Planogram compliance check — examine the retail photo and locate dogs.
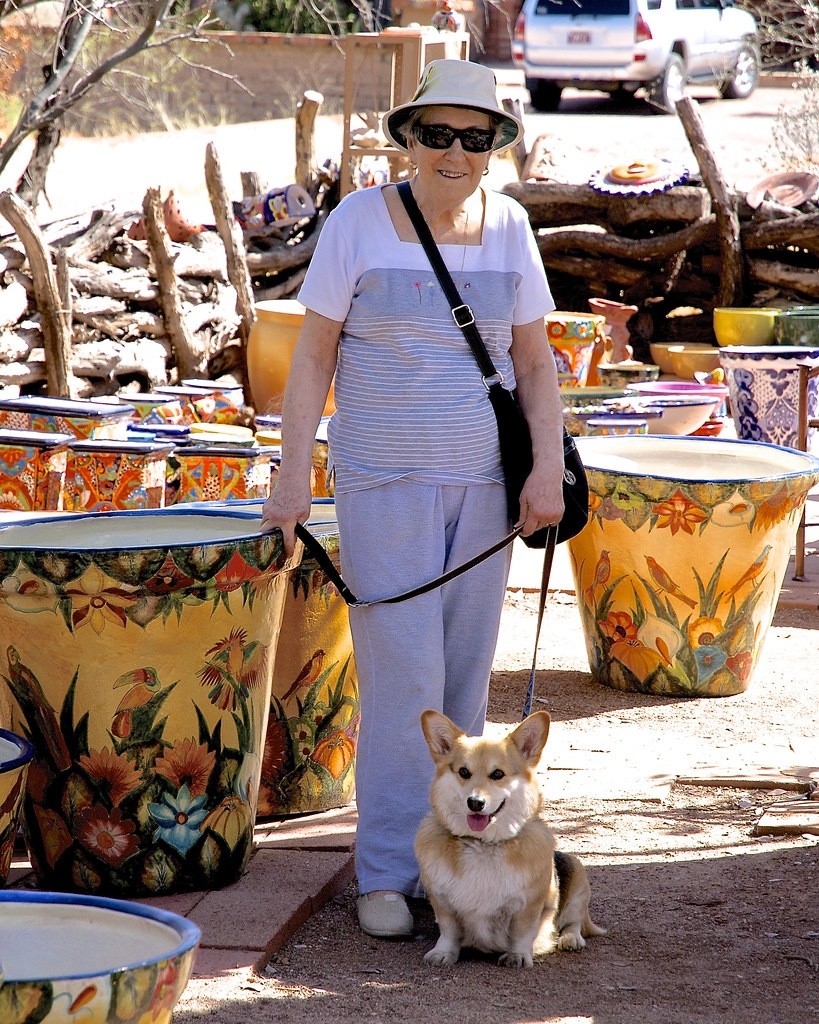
[414,710,607,970]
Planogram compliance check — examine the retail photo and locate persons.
[259,59,564,935]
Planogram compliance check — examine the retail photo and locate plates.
[746,170,819,210]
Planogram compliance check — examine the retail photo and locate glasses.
[412,123,497,153]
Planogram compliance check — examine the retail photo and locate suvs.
[510,0,761,113]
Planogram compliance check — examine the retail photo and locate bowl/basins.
[561,342,729,437]
[774,311,819,347]
[713,308,782,346]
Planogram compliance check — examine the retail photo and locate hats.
[381,58,526,157]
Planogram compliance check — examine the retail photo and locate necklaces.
[409,175,470,297]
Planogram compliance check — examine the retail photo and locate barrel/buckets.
[163,499,361,816]
[566,434,819,698]
[0,890,202,1024]
[0,728,33,890]
[719,346,819,453]
[0,509,308,899]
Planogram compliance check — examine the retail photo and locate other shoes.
[356,893,414,938]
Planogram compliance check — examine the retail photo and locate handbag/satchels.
[486,384,590,549]
